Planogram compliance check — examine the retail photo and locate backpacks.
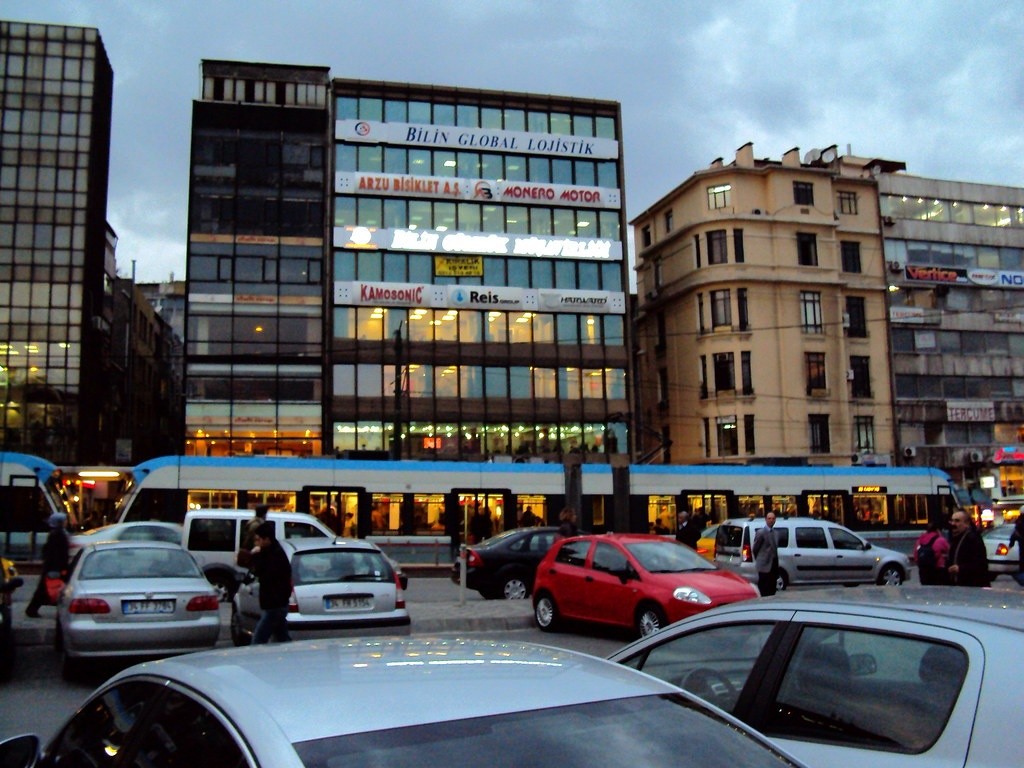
[916,535,939,571]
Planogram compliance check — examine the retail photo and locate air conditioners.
[852,453,862,465]
[904,446,916,457]
[752,208,765,215]
[970,451,983,462]
[842,314,850,327]
[886,214,896,225]
[846,369,854,380]
[891,261,904,270]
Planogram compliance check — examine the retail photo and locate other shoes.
[25,609,41,618]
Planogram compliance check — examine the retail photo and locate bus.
[0,447,975,578]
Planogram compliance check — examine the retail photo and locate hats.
[48,511,68,527]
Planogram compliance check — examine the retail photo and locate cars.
[66,520,188,566]
[532,534,764,641]
[452,525,585,603]
[0,632,814,768]
[56,538,221,667]
[983,523,1024,590]
[229,540,413,647]
[606,585,1022,768]
[696,514,917,594]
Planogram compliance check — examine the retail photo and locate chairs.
[875,644,967,746]
[786,645,859,741]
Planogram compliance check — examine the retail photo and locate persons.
[25,512,72,618]
[914,518,951,585]
[468,507,494,545]
[693,500,883,529]
[516,506,545,527]
[650,518,677,535]
[675,511,701,552]
[244,504,272,586]
[752,511,779,597]
[248,520,293,645]
[1006,505,1024,585]
[446,521,461,564]
[60,470,133,530]
[945,508,991,587]
[554,506,579,544]
